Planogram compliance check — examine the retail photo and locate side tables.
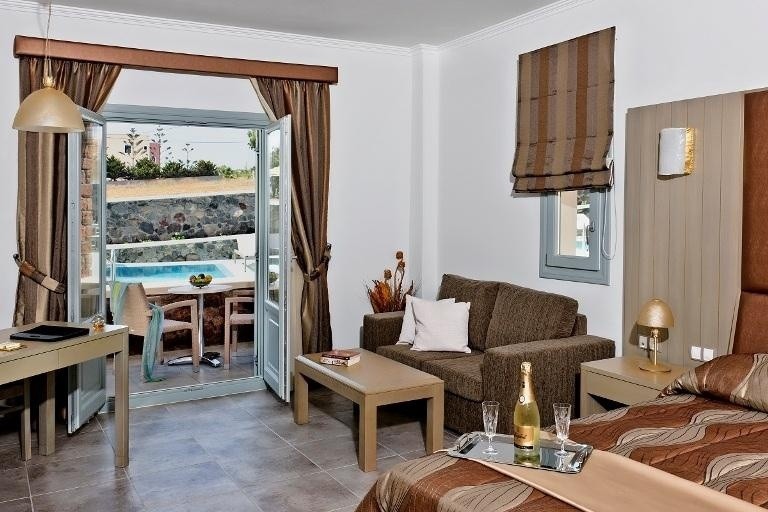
[580,355,691,419]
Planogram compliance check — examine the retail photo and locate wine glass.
[552,403,571,457]
[482,401,500,454]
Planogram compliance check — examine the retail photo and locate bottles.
[513,362,540,465]
[93,314,105,331]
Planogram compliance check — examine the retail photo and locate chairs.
[224,290,255,370]
[109,281,199,372]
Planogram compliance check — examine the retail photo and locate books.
[320,350,361,367]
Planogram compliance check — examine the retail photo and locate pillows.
[410,300,471,353]
[484,282,578,349]
[437,273,498,352]
[657,353,768,412]
[396,294,456,347]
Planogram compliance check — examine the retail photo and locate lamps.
[12,0,85,134]
[657,127,695,177]
[636,299,673,372]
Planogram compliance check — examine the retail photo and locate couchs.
[363,311,615,439]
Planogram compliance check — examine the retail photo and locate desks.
[168,284,232,368]
[0,320,128,468]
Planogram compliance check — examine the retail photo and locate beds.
[356,294,768,512]
